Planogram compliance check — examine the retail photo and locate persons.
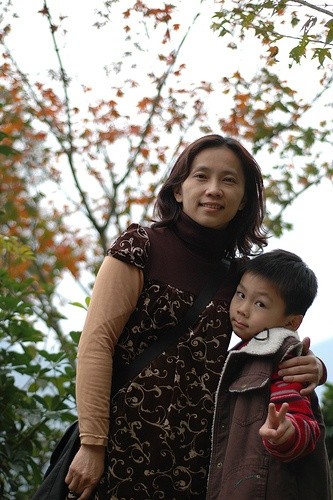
[204,248,333,500]
[29,134,328,500]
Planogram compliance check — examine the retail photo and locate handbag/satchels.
[30,419,81,500]
